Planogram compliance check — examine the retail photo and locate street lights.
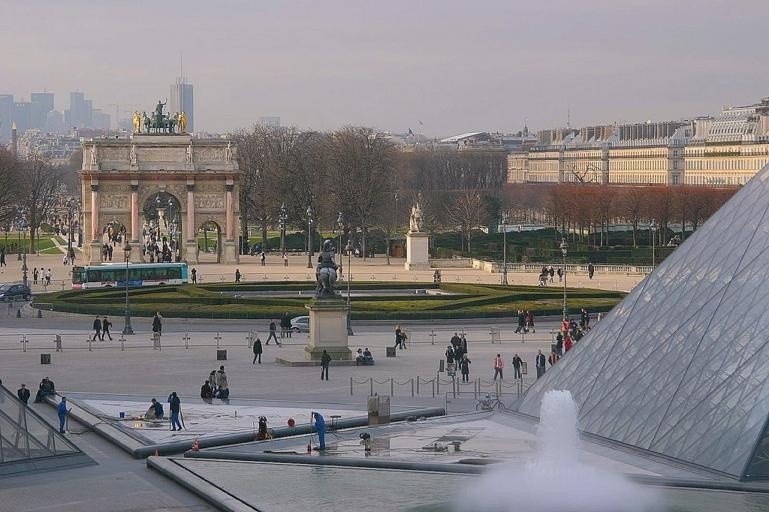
[394,192,399,234]
[156,194,162,241]
[559,238,568,320]
[361,217,367,261]
[477,193,482,228]
[306,205,314,269]
[281,201,285,259]
[122,239,134,335]
[500,217,508,286]
[168,197,174,246]
[336,212,342,275]
[10,122,18,157]
[345,239,353,337]
[650,219,657,272]
[2,196,82,285]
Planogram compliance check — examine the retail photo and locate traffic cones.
[191,437,199,452]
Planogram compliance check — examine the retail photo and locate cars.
[0,282,32,301]
[289,315,309,334]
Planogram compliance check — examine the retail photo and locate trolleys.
[257,416,274,440]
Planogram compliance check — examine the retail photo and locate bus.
[70,262,188,289]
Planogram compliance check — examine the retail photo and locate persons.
[536,306,590,366]
[369,245,377,258]
[150,392,183,432]
[155,98,167,114]
[433,270,440,282]
[598,312,603,322]
[92,315,114,341]
[312,412,326,450]
[538,262,594,286]
[514,310,536,333]
[18,376,70,433]
[260,251,265,265]
[151,312,163,336]
[1,226,200,285]
[201,366,230,406]
[284,253,288,267]
[256,417,272,440]
[321,323,523,383]
[235,269,242,282]
[191,438,198,451]
[316,240,342,274]
[253,311,292,364]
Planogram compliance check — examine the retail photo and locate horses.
[142,111,179,134]
[319,267,337,296]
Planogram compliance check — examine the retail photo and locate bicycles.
[475,392,505,412]
